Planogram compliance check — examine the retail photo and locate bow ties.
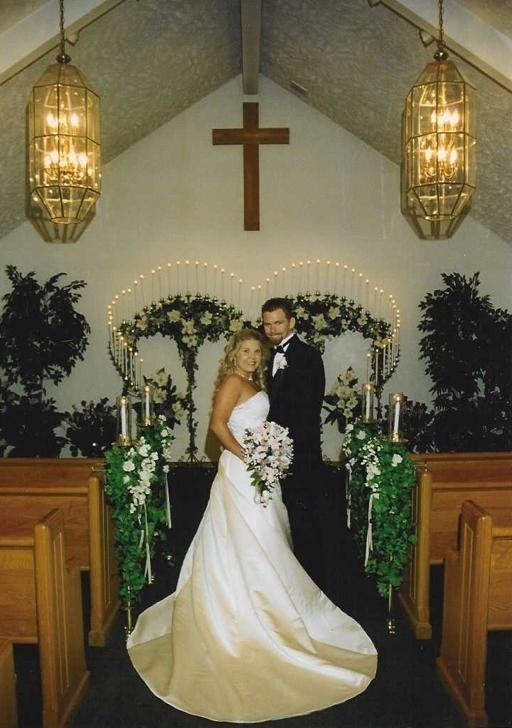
[270,345,285,355]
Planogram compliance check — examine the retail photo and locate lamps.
[26,0,101,223]
[404,0,475,222]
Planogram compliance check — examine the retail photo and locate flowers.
[106,417,171,606]
[346,420,415,597]
[242,422,295,505]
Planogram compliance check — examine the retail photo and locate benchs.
[397,451,512,728]
[0,455,124,728]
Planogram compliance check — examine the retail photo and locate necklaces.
[235,372,258,389]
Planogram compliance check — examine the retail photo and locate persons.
[262,297,327,578]
[125,329,380,723]
